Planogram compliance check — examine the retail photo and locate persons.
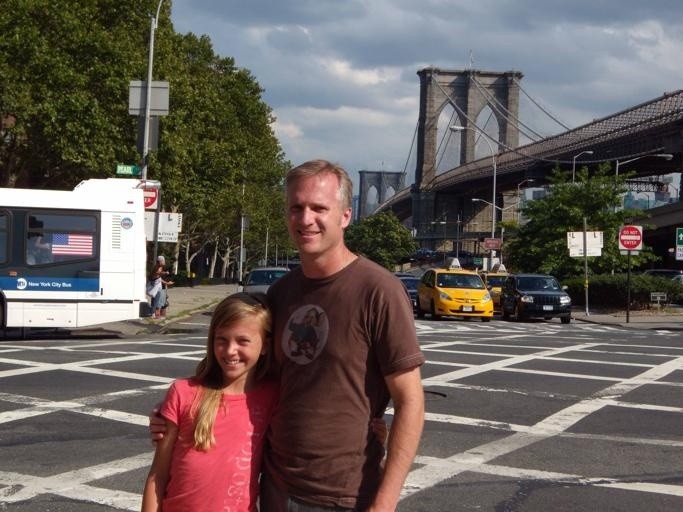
[142,293,387,512]
[150,265,175,319]
[149,163,425,511]
[35,236,63,265]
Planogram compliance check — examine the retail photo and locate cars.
[500,274,572,324]
[238,267,292,295]
[416,257,495,323]
[395,274,415,278]
[396,277,422,314]
[479,264,512,315]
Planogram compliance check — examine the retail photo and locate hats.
[156,255,164,263]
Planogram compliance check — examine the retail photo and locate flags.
[51,233,93,257]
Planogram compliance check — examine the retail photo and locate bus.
[0,178,144,339]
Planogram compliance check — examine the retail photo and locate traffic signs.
[675,228,683,261]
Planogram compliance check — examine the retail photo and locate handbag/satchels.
[146,281,160,298]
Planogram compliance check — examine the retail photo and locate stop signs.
[144,188,158,210]
[618,225,643,250]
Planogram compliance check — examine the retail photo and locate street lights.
[516,178,535,225]
[614,153,673,213]
[470,196,531,264]
[448,124,497,259]
[572,150,593,183]
